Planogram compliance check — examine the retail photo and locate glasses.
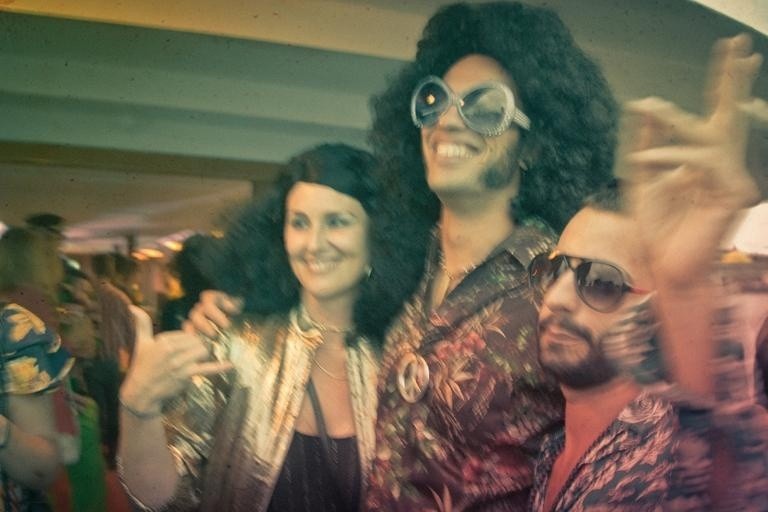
[408,73,532,138]
[525,250,656,315]
